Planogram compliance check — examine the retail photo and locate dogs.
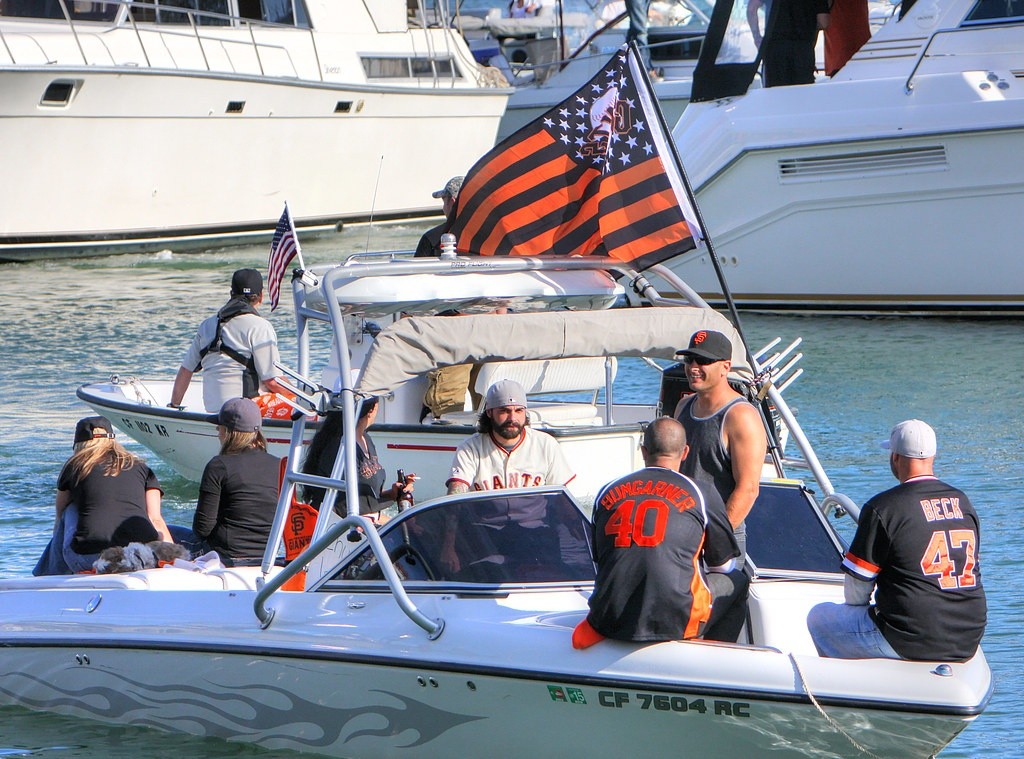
[92,541,192,575]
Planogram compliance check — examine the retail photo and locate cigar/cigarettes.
[408,476,421,479]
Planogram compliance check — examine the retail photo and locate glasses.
[215,426,226,430]
[683,352,721,366]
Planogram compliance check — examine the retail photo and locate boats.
[407,0,901,147]
[618,0,1024,317]
[0,0,515,261]
[76,260,804,514]
[0,233,994,759]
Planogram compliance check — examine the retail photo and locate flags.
[433,44,703,280]
[267,207,297,312]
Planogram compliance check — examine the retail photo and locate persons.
[166,397,281,567]
[440,379,594,575]
[400,176,508,425]
[586,329,768,641]
[298,368,414,533]
[746,0,831,88]
[625,0,665,83]
[167,268,318,422]
[32,417,173,576]
[806,418,988,663]
[508,0,540,18]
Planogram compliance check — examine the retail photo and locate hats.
[232,268,264,295]
[331,368,392,408]
[433,176,464,199]
[675,329,732,362]
[879,419,935,459]
[72,415,115,449]
[484,380,528,409]
[205,398,263,432]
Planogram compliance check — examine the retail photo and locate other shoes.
[647,71,663,81]
